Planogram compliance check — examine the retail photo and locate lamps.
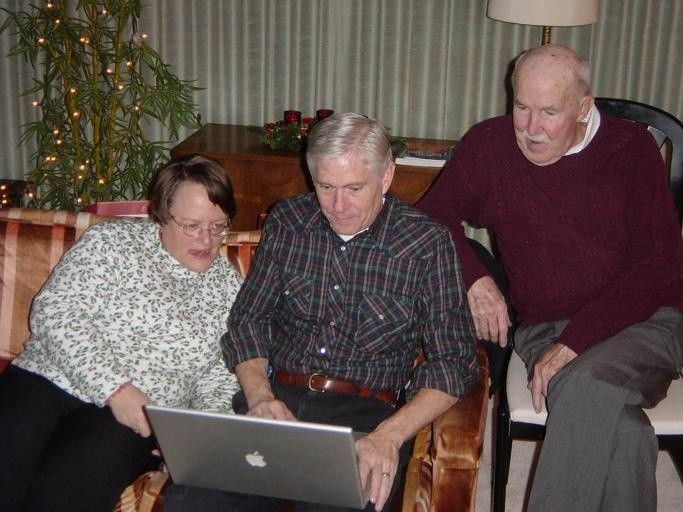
[486,0,600,48]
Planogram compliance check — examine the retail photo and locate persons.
[1,154,244,512]
[418,43,683,510]
[164,111,481,511]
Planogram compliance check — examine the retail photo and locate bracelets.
[104,380,132,405]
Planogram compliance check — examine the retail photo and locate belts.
[274,368,399,408]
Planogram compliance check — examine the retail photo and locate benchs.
[1,209,488,512]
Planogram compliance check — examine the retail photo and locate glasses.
[167,211,231,239]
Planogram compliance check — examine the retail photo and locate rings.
[132,427,141,434]
[379,470,392,477]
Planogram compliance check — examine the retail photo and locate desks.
[171,122,457,229]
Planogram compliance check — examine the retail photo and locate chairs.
[488,99,683,512]
[83,195,271,227]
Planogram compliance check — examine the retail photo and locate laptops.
[144,404,370,512]
